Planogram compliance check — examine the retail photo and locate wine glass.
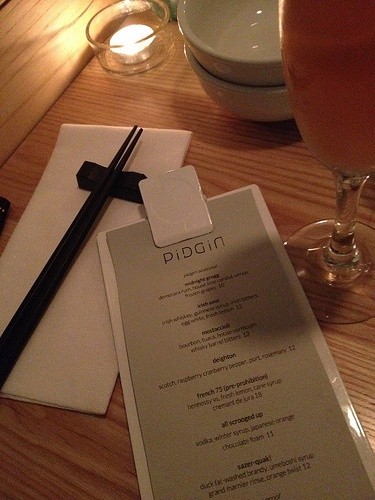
[277,1,374,325]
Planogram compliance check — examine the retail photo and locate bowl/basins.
[177,1,286,86]
[181,42,297,121]
[85,0,174,77]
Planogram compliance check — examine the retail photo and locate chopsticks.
[1,124,143,388]
